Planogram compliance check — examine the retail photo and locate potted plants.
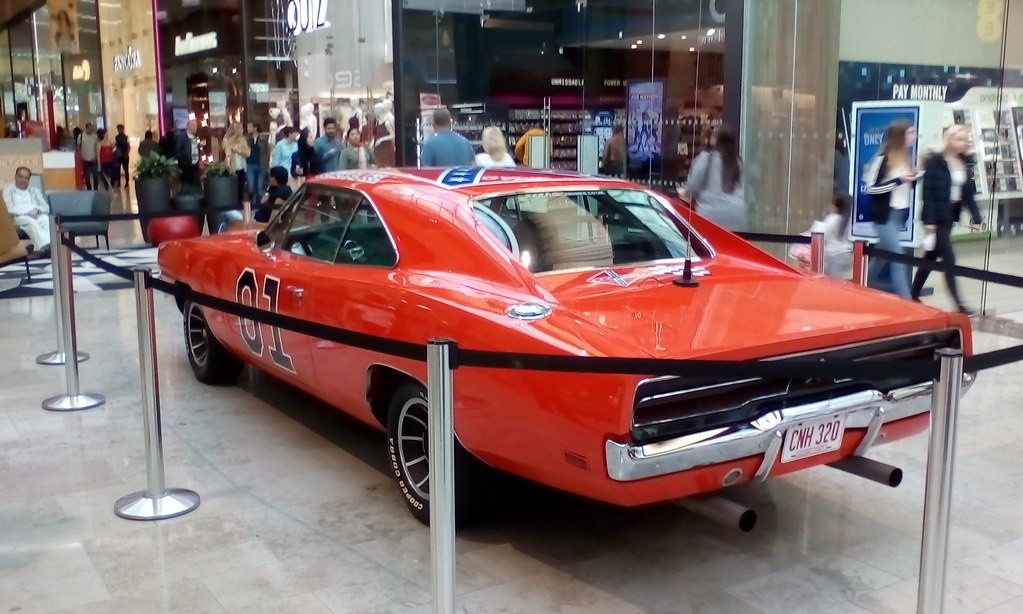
[132,150,183,243]
[203,162,239,235]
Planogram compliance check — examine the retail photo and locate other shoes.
[25,243,52,260]
[958,306,978,319]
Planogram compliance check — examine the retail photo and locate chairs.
[48,191,112,251]
[0,192,32,281]
[15,193,52,260]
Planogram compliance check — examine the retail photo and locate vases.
[171,194,204,211]
[147,211,202,246]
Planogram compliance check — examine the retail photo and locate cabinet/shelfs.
[416,118,508,168]
[509,108,593,173]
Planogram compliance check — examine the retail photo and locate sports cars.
[158,165,975,525]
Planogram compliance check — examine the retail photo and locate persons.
[2,110,50,153]
[601,125,626,176]
[272,126,301,193]
[474,126,517,168]
[77,123,99,191]
[813,193,855,281]
[69,128,81,150]
[56,126,69,149]
[861,120,922,304]
[312,118,350,223]
[2,167,57,254]
[267,96,397,172]
[258,166,294,222]
[514,122,554,168]
[243,122,266,208]
[910,125,984,317]
[420,108,478,167]
[96,129,117,190]
[630,106,661,155]
[158,129,176,159]
[175,120,203,191]
[138,130,159,160]
[220,121,252,210]
[339,128,375,224]
[292,126,323,226]
[677,123,744,234]
[113,125,130,188]
